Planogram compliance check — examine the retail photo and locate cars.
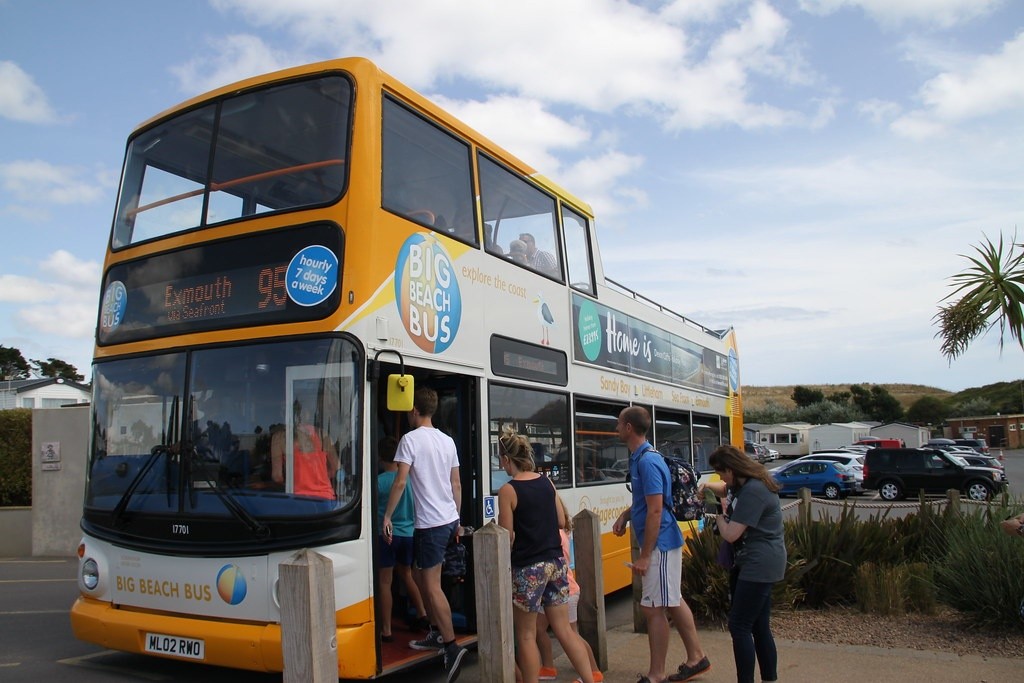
[810,435,991,457]
[742,441,780,464]
[788,453,865,485]
[764,460,858,500]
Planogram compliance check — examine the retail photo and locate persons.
[271,391,339,500]
[698,445,787,683]
[382,388,469,683]
[612,406,714,683]
[376,436,430,643]
[498,430,604,683]
[452,206,557,277]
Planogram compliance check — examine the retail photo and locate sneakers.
[443,641,470,683]
[572,670,604,683]
[636,670,670,683]
[667,656,713,682]
[538,664,558,681]
[409,625,446,651]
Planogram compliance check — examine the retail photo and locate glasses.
[500,434,527,452]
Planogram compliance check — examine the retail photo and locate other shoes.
[410,615,429,633]
[380,630,394,642]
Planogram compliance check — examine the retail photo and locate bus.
[67,56,742,681]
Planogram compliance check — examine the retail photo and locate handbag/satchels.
[717,539,735,572]
[443,543,470,576]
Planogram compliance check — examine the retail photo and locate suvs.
[952,454,1010,485]
[862,448,1009,502]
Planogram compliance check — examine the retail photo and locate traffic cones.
[997,448,1006,460]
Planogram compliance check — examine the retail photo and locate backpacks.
[639,447,706,522]
